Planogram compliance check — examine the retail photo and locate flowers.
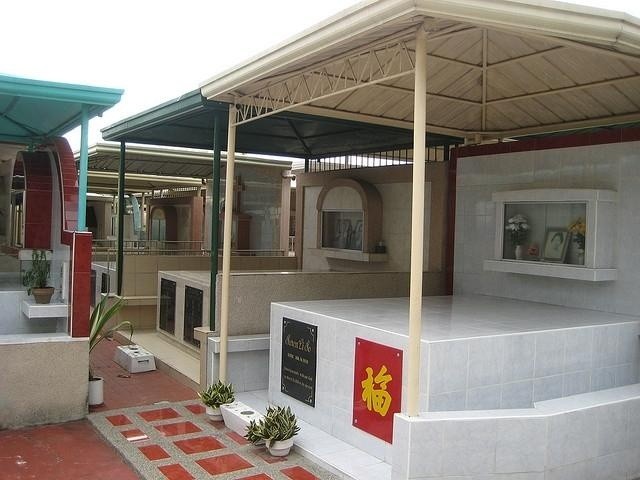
[504,213,530,245]
[568,217,586,250]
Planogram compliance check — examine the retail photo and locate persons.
[543,230,565,258]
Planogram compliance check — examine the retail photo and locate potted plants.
[197,380,235,421]
[244,405,301,456]
[88,291,134,405]
[22,249,54,304]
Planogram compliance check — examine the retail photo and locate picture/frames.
[334,219,350,249]
[351,220,362,250]
[538,228,571,263]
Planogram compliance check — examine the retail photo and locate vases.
[578,249,584,265]
[515,245,523,260]
